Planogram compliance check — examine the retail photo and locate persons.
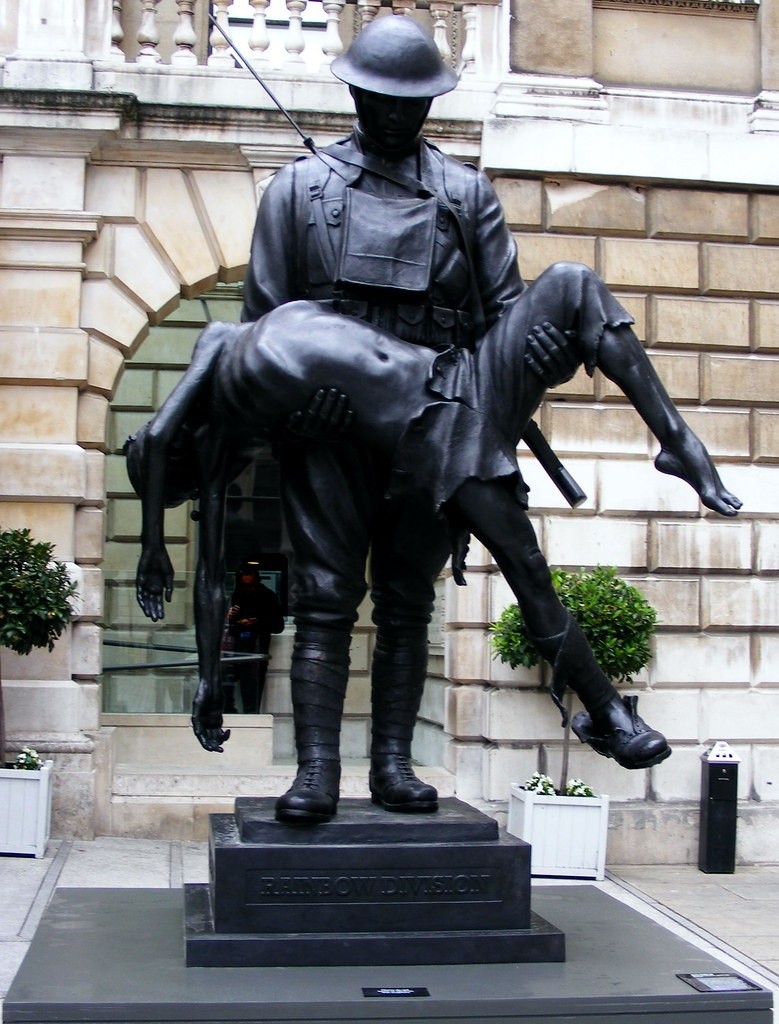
[124,261,744,769]
[240,15,581,820]
[225,567,285,713]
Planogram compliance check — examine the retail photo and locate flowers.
[13,746,45,771]
[525,771,595,798]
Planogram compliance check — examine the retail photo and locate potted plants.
[486,563,665,880]
[0,527,86,862]
